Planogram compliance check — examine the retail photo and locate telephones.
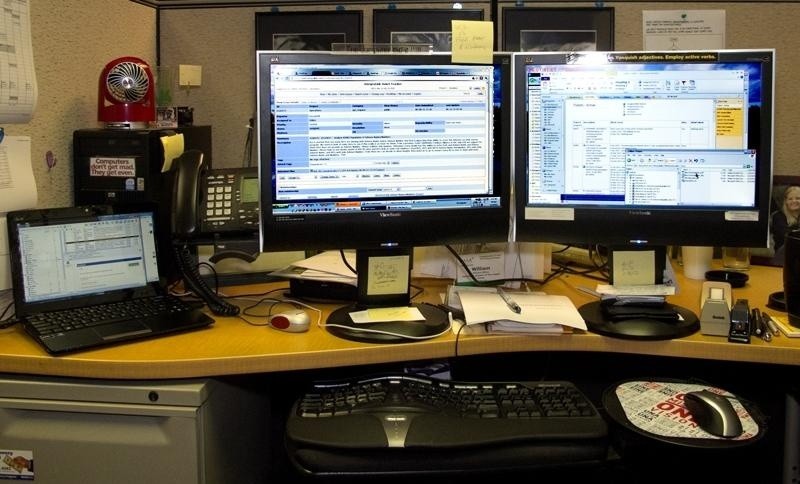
[169,148,259,249]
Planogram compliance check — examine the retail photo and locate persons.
[771,185,800,255]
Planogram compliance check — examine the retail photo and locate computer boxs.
[68,123,214,289]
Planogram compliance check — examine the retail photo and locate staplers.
[728,299,751,343]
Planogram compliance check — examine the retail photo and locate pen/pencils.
[496,286,521,313]
[751,308,780,341]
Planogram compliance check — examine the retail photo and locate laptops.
[6,200,216,358]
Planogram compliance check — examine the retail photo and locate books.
[771,316,800,337]
[485,291,574,337]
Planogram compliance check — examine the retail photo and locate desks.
[2,250,800,484]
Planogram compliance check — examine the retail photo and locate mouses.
[267,308,311,334]
[684,388,743,438]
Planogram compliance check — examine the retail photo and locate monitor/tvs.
[252,47,514,346]
[510,45,778,343]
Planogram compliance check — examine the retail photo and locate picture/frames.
[500,4,617,53]
[744,174,800,268]
[371,8,485,53]
[253,10,365,112]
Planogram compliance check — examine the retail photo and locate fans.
[91,55,156,129]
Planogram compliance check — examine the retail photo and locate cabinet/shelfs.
[3,379,270,484]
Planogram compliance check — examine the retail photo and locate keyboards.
[285,375,608,448]
